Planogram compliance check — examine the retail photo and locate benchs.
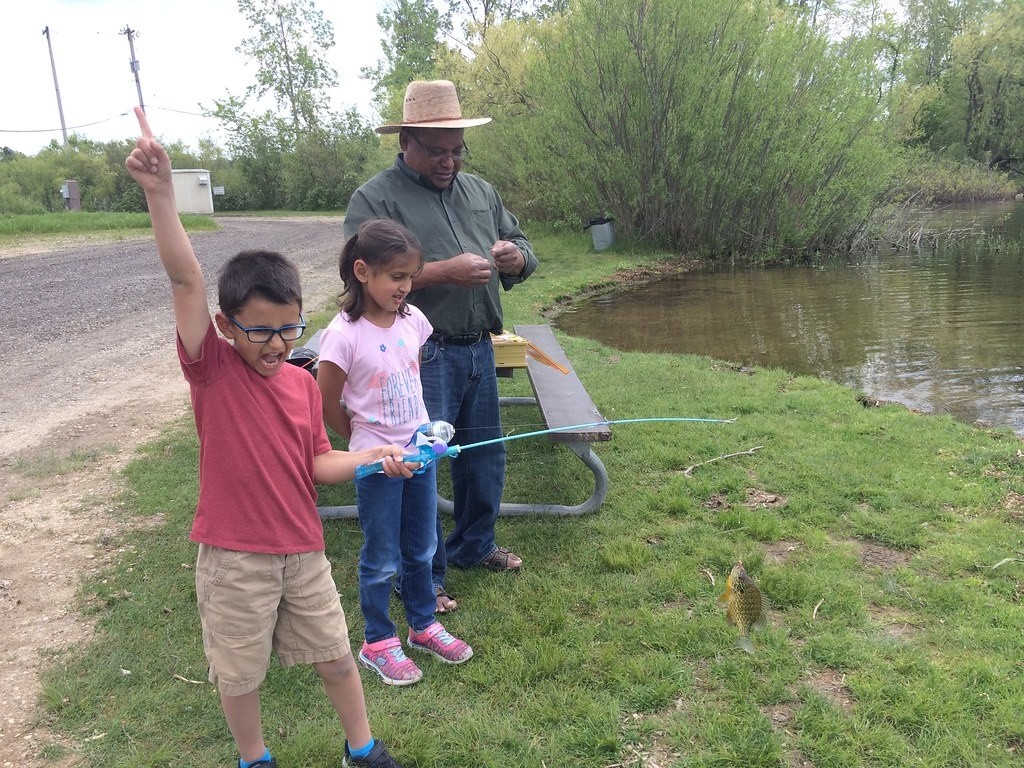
[514,323,612,443]
[286,328,325,376]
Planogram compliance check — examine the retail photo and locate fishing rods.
[354,417,738,478]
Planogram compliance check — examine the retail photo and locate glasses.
[226,312,307,343]
[406,129,469,162]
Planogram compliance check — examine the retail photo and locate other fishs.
[718,567,769,653]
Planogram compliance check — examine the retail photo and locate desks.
[312,360,514,518]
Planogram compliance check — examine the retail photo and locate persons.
[127,107,422,768]
[344,80,539,614]
[317,220,474,687]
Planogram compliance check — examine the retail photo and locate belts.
[427,330,489,346]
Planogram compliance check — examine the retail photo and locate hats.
[374,80,492,134]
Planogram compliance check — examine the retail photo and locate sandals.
[481,545,520,573]
[394,584,458,615]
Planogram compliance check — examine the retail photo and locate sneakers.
[358,636,423,687]
[238,757,278,768]
[406,621,473,665]
[342,739,403,768]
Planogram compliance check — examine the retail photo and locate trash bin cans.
[589,218,615,249]
